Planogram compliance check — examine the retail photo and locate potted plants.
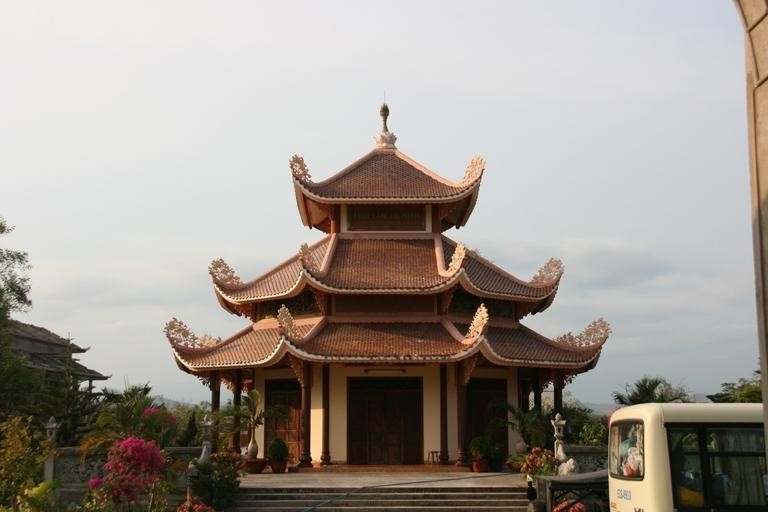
[215,389,293,473]
[468,436,492,473]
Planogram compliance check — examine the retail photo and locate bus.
[607,402,768,512]
